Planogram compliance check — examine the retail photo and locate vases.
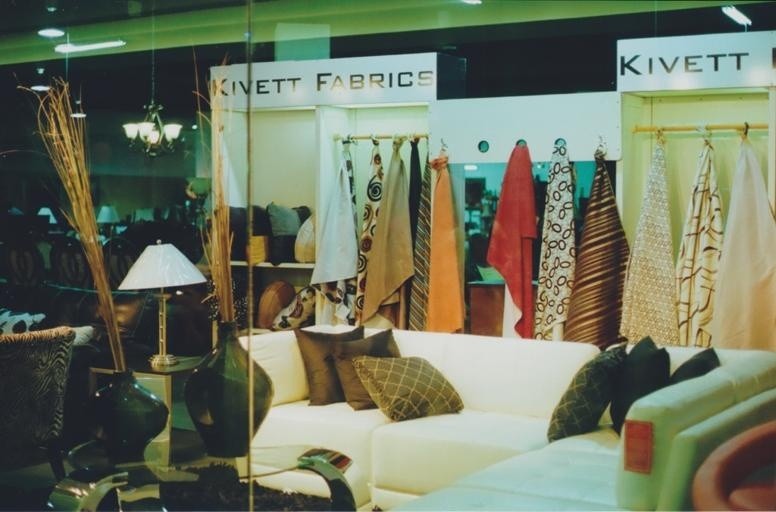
[184,319,275,457]
[90,368,168,469]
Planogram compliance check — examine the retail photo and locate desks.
[470,281,538,337]
[46,436,377,512]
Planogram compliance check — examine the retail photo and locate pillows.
[547,338,719,444]
[230,203,315,265]
[256,279,314,329]
[292,325,463,422]
[0,327,77,481]
[63,326,96,344]
[1,307,47,333]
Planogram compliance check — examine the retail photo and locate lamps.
[120,0,184,158]
[94,204,121,254]
[118,238,208,369]
[37,207,60,240]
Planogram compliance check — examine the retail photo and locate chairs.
[691,421,776,512]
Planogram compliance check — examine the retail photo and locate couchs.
[237,324,775,512]
[39,281,149,347]
